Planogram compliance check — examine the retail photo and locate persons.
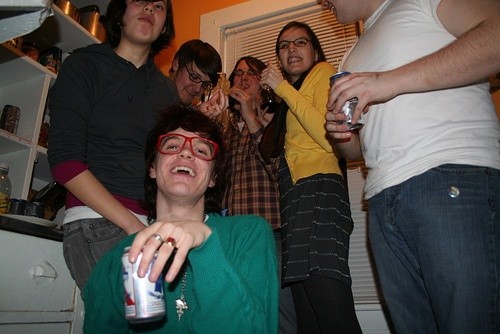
[168,39,222,105]
[221,57,280,229]
[259,22,364,334]
[321,0,500,334]
[82,109,278,334]
[48,0,229,290]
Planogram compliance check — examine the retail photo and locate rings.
[165,238,176,247]
[152,234,163,244]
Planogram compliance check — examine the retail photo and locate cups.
[233,82,251,99]
[257,86,281,111]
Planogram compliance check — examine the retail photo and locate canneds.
[329,71,364,130]
[121,246,165,325]
[0,104,20,134]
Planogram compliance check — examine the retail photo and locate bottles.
[0,163,12,214]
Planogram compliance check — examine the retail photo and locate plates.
[3,215,57,228]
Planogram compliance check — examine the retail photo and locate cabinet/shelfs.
[0,1,109,334]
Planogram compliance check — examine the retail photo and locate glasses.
[277,36,313,50]
[156,134,217,160]
[184,65,215,90]
